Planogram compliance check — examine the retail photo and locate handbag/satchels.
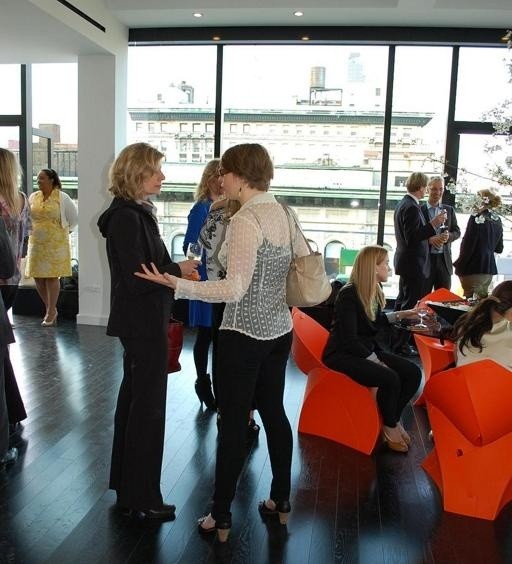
[324,240,346,278]
[63,259,78,290]
[280,201,332,308]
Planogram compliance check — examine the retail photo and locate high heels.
[382,426,408,452]
[41,309,58,326]
[195,378,215,408]
[198,516,231,542]
[258,499,291,524]
[402,432,410,444]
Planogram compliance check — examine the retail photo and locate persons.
[0,214,28,475]
[96,142,203,522]
[452,189,504,304]
[0,147,31,312]
[320,244,430,453]
[393,172,448,311]
[133,142,310,543]
[197,196,260,443]
[182,158,224,412]
[419,175,462,302]
[23,168,80,328]
[448,279,512,376]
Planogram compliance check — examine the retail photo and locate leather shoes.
[130,505,176,520]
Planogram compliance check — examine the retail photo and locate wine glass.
[186,243,204,261]
[414,300,430,329]
[438,209,449,229]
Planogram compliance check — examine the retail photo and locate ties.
[418,204,427,225]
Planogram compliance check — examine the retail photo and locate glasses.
[218,168,230,176]
[429,187,444,193]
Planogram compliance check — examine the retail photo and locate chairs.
[414,288,473,405]
[419,358,511,522]
[291,304,383,455]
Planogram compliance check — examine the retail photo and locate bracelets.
[394,311,402,323]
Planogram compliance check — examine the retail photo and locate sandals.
[244,419,260,442]
